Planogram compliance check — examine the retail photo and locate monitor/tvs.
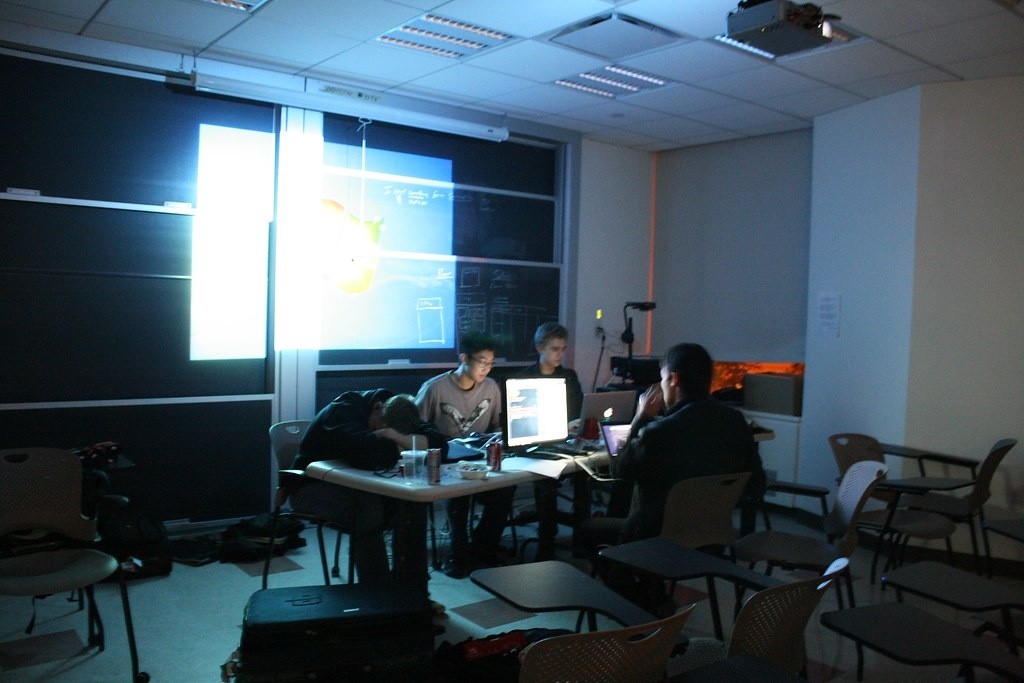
[501,375,568,461]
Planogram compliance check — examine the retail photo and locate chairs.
[429,433,1023,683]
[0,447,150,683]
[262,421,354,589]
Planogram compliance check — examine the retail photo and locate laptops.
[597,417,644,460]
[566,391,636,449]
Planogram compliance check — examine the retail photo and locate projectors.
[609,356,662,384]
[727,0,832,57]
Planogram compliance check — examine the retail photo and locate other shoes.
[447,556,464,577]
[424,598,444,616]
[475,542,505,565]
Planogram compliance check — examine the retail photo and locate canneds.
[427,448,441,485]
[487,442,501,472]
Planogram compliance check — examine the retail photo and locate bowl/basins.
[456,465,493,479]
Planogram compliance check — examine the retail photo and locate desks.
[307,425,775,584]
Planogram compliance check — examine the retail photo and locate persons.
[575,342,767,643]
[514,322,592,562]
[290,388,449,636]
[413,333,517,575]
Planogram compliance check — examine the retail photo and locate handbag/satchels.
[222,513,305,561]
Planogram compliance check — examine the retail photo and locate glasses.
[467,356,496,368]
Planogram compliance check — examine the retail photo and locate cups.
[401,450,427,485]
